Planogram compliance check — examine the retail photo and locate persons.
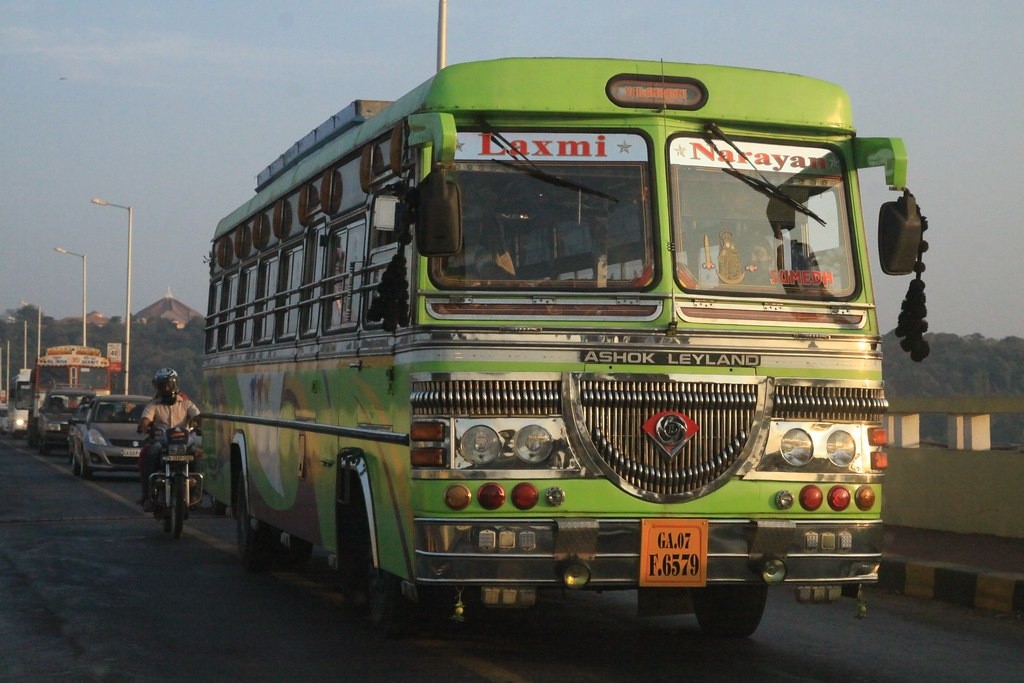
[137,368,202,505]
[103,407,115,421]
[121,403,132,417]
[82,398,91,403]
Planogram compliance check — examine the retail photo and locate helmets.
[152,367,179,404]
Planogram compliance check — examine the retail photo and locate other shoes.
[143,498,153,512]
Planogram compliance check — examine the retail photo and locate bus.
[25,345,112,403]
[9,368,41,440]
[200,55,931,637]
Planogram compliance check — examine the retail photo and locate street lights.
[52,246,87,347]
[91,197,132,396]
[19,300,41,360]
[9,316,28,371]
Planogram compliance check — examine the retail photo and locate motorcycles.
[143,424,204,541]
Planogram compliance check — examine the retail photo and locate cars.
[0,404,12,433]
[68,393,165,481]
[37,390,98,451]
